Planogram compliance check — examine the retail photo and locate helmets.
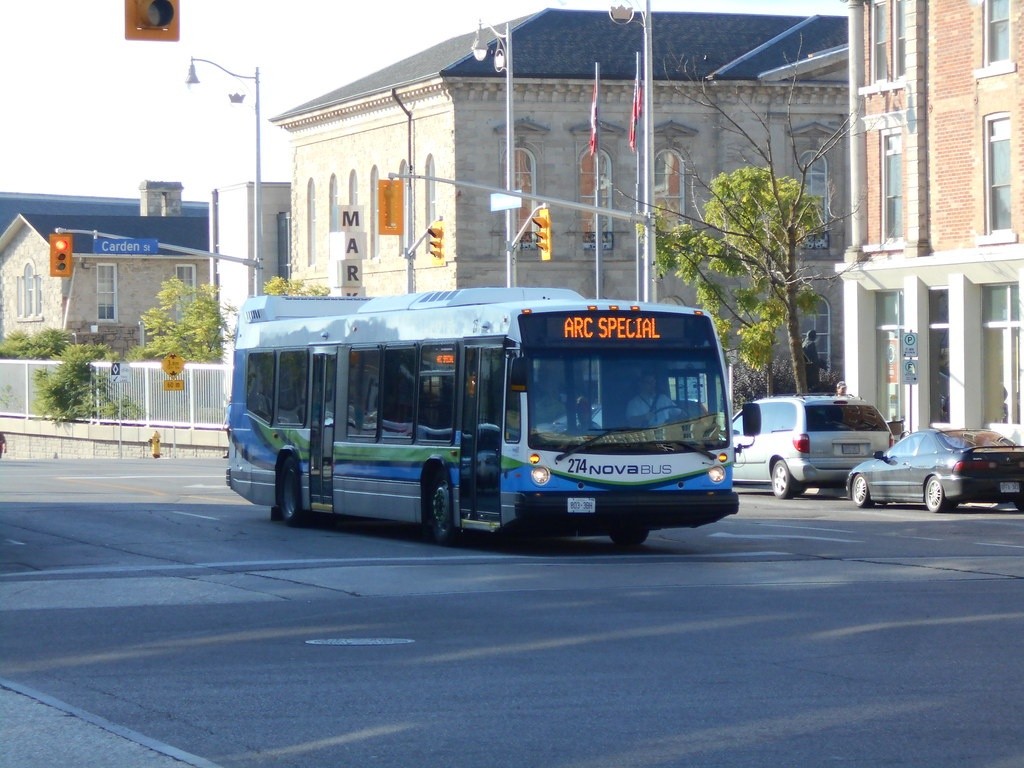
[576,397,589,413]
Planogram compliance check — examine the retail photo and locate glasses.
[645,380,657,386]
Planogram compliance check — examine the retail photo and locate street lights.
[183,58,273,300]
[473,19,521,289]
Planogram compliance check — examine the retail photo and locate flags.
[627,72,642,152]
[589,76,598,157]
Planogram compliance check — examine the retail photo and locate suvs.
[725,392,898,500]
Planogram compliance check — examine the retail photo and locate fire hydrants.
[148,430,164,459]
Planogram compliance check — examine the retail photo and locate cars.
[847,426,1024,512]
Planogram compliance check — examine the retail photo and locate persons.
[802,330,819,385]
[836,381,848,395]
[625,371,685,428]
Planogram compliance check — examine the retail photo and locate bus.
[225,287,741,556]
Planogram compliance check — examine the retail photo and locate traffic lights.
[533,208,553,261]
[124,0,180,41]
[49,233,74,279]
[429,217,446,267]
